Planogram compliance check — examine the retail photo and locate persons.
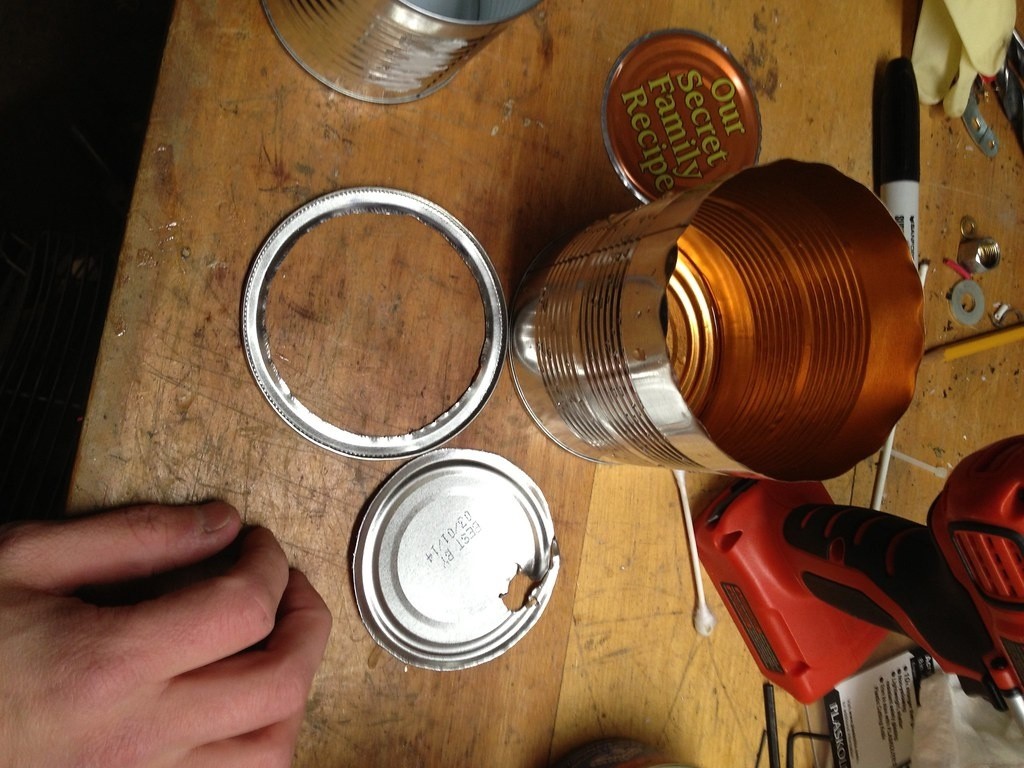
[0,501,333,768]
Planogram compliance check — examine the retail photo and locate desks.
[67,0,1024,768]
